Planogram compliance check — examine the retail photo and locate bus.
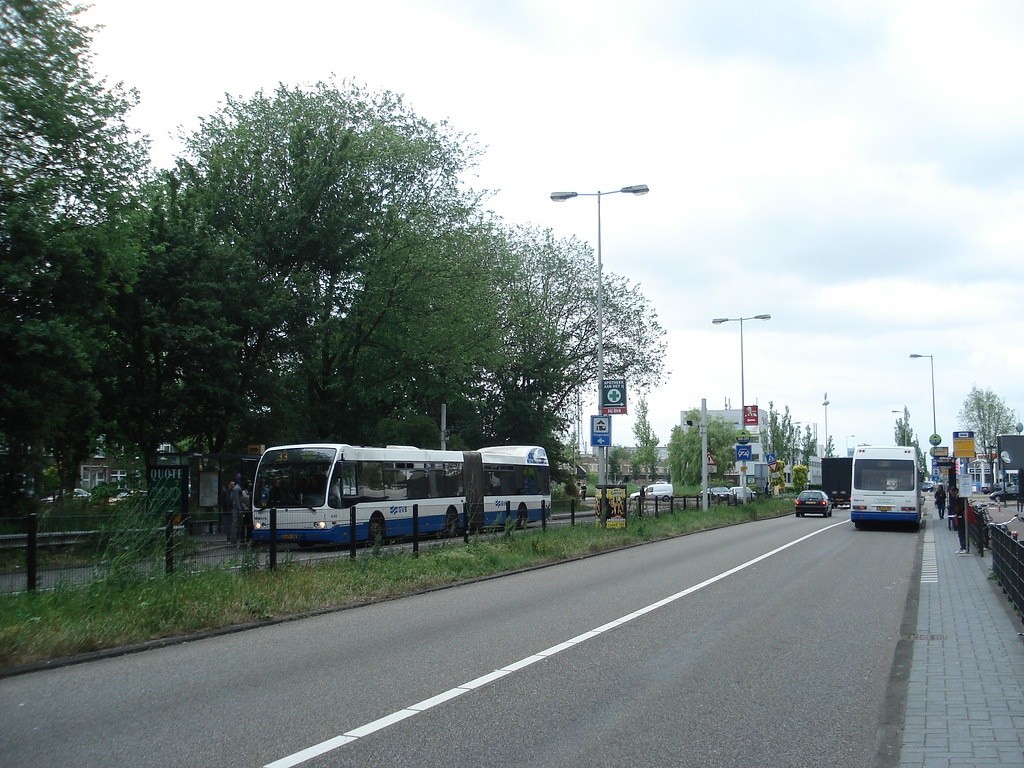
[850,443,926,532]
[251,442,552,548]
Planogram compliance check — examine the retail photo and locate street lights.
[909,353,937,446]
[712,314,771,504]
[821,391,830,458]
[549,184,650,526]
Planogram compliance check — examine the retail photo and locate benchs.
[190,520,218,534]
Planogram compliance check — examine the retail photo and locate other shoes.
[959,549,966,553]
[955,549,962,554]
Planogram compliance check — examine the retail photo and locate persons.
[576,483,587,500]
[222,473,252,545]
[947,487,970,554]
[764,482,770,499]
[640,485,646,510]
[256,476,305,504]
[935,485,946,519]
[1007,483,1011,487]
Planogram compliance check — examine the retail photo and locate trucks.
[820,458,853,509]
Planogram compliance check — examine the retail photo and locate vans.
[629,483,674,502]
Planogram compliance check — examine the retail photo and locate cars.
[793,490,835,518]
[989,484,1019,502]
[41,488,148,509]
[921,480,949,493]
[728,486,758,505]
[700,487,731,505]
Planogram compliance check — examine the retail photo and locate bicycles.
[968,499,1024,545]
